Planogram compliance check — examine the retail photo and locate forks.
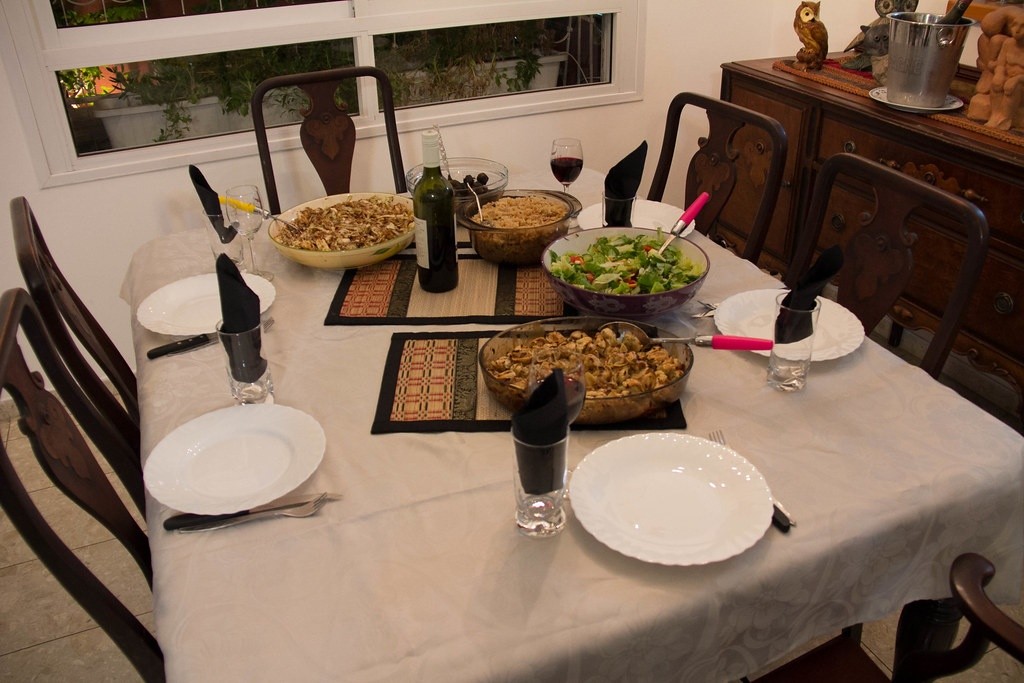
[177,492,327,534]
[167,317,274,356]
[709,428,796,528]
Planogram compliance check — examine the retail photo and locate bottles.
[935,0,973,24]
[413,129,460,294]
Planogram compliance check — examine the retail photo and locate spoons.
[595,320,772,352]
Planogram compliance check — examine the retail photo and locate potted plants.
[89,20,571,151]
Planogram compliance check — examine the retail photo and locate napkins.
[217,252,268,384]
[188,164,238,244]
[775,244,846,344]
[512,368,567,496]
[605,141,647,227]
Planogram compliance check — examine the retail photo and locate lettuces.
[547,227,703,295]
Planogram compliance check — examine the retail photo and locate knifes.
[146,332,217,359]
[690,311,716,319]
[161,493,341,530]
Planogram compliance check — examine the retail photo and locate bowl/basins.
[267,157,709,426]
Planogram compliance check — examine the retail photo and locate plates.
[142,404,327,516]
[569,432,773,567]
[137,271,276,336]
[714,288,865,361]
[869,87,964,114]
[578,199,695,238]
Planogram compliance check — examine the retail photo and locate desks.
[118,162,1024,683]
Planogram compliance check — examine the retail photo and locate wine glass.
[548,137,583,193]
[225,185,274,281]
[529,351,588,499]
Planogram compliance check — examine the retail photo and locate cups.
[766,293,822,392]
[512,425,569,538]
[216,319,275,404]
[203,204,244,264]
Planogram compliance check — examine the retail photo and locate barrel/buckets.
[886,12,977,107]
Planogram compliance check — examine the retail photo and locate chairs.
[783,153,990,381]
[0,195,166,683]
[648,92,789,265]
[251,66,407,214]
[749,552,1024,683]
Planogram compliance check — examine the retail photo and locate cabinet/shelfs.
[714,50,1024,419]
